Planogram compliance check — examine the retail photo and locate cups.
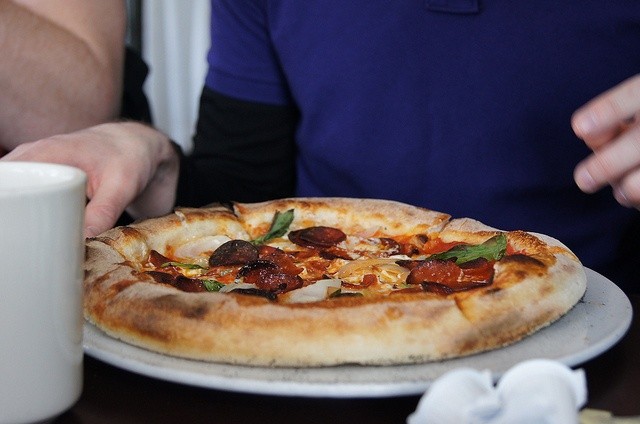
[0,160,86,423]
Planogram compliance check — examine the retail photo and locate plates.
[82,264,634,398]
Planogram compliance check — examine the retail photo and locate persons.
[0,1,640,242]
[1,1,126,152]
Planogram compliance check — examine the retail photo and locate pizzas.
[80,192,589,368]
